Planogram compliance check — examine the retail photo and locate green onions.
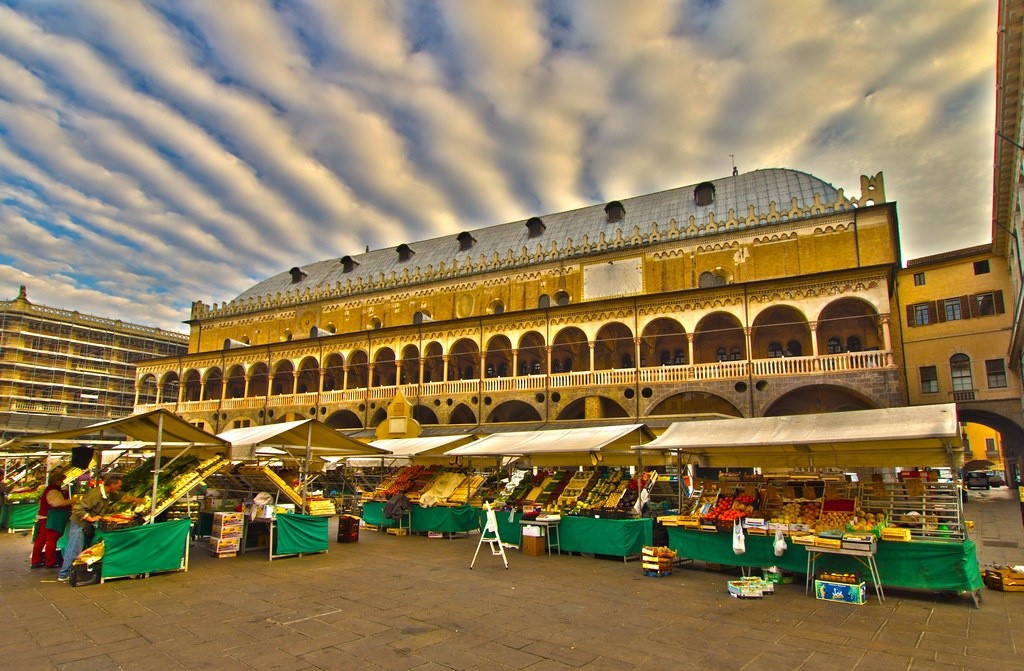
[108,484,173,514]
[586,478,606,501]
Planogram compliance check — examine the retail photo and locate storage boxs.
[814,579,868,606]
[209,512,244,554]
[727,569,798,600]
[522,535,546,557]
[987,567,1024,593]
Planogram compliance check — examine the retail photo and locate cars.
[967,470,1001,490]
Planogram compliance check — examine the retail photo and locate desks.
[479,509,653,564]
[517,520,562,558]
[361,502,483,541]
[668,525,985,608]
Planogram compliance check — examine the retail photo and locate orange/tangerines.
[820,511,851,529]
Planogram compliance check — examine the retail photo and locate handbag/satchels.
[46,508,69,536]
[733,516,745,554]
[773,524,788,556]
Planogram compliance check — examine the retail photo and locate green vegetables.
[504,472,542,500]
[101,455,194,498]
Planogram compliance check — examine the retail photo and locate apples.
[771,502,821,525]
[702,493,755,529]
[820,572,855,583]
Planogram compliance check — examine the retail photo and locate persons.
[58,476,144,581]
[0,468,14,531]
[29,473,77,568]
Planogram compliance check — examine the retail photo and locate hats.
[48,472,67,485]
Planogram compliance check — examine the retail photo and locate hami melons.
[852,511,884,530]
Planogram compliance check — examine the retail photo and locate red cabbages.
[618,497,636,507]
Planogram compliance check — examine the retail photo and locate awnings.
[630,402,965,514]
[0,409,480,524]
[443,424,673,501]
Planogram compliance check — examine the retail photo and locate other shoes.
[30,561,45,569]
[45,563,61,568]
[58,575,81,582]
